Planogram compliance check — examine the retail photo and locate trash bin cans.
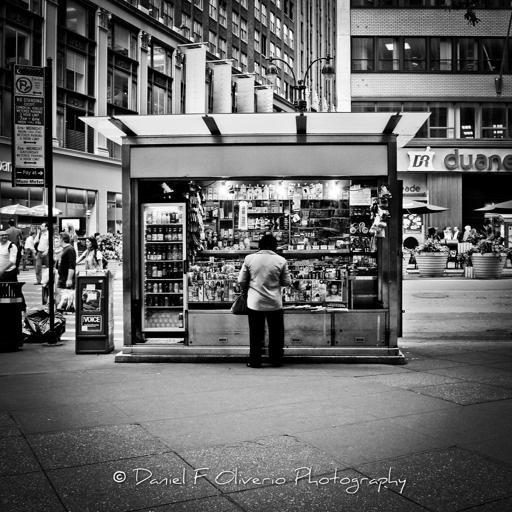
[0,281,27,351]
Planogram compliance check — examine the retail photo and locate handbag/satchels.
[94,249,108,269]
[229,294,248,316]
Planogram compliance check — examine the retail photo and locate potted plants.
[464,239,512,279]
[402,247,412,276]
[413,238,450,277]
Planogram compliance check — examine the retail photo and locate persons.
[0,218,106,314]
[237,233,293,368]
[442,224,478,269]
[328,282,343,303]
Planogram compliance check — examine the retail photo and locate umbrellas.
[30,202,64,224]
[0,203,36,227]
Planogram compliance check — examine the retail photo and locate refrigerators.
[139,200,188,336]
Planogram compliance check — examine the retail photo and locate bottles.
[145,206,183,329]
[205,229,252,251]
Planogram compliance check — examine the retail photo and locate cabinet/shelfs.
[334,309,390,347]
[265,309,333,348]
[201,200,350,254]
[185,310,250,346]
[187,259,350,304]
[350,276,382,313]
